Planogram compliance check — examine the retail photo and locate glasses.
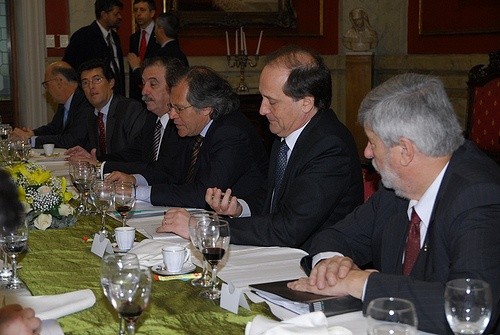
[42,79,62,88]
[81,77,107,88]
[167,103,194,114]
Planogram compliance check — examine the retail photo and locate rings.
[165,218,167,224]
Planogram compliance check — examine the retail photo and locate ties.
[140,30,147,63]
[272,139,290,211]
[63,109,68,130]
[403,207,422,276]
[105,33,120,94]
[98,112,106,155]
[185,135,204,185]
[152,120,162,163]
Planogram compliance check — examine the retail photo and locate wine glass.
[188,211,220,287]
[109,264,152,335]
[0,220,29,291]
[196,219,230,300]
[100,252,139,335]
[69,161,136,238]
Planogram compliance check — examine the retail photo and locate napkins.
[245,312,354,335]
[121,239,190,268]
[5,288,96,321]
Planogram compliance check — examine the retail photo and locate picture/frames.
[166,0,298,31]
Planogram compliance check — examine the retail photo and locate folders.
[248,279,363,316]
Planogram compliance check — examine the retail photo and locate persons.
[0,168,41,335]
[155,48,363,252]
[125,0,162,107]
[287,73,500,335]
[128,11,188,76]
[68,51,188,186]
[62,60,155,169]
[105,65,267,208]
[64,0,125,91]
[11,61,97,151]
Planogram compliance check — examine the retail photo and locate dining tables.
[0,149,429,335]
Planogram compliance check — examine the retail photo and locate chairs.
[465,64,500,165]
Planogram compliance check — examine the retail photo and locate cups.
[366,297,418,335]
[162,246,189,273]
[43,144,55,157]
[0,115,12,140]
[0,138,30,165]
[115,226,136,249]
[444,279,492,335]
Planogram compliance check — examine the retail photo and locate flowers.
[4,162,78,231]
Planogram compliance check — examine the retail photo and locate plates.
[151,261,196,276]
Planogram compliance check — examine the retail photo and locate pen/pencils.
[40,153,59,155]
[164,212,211,217]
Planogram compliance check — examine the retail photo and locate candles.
[255,31,263,55]
[235,26,247,55]
[225,31,231,55]
